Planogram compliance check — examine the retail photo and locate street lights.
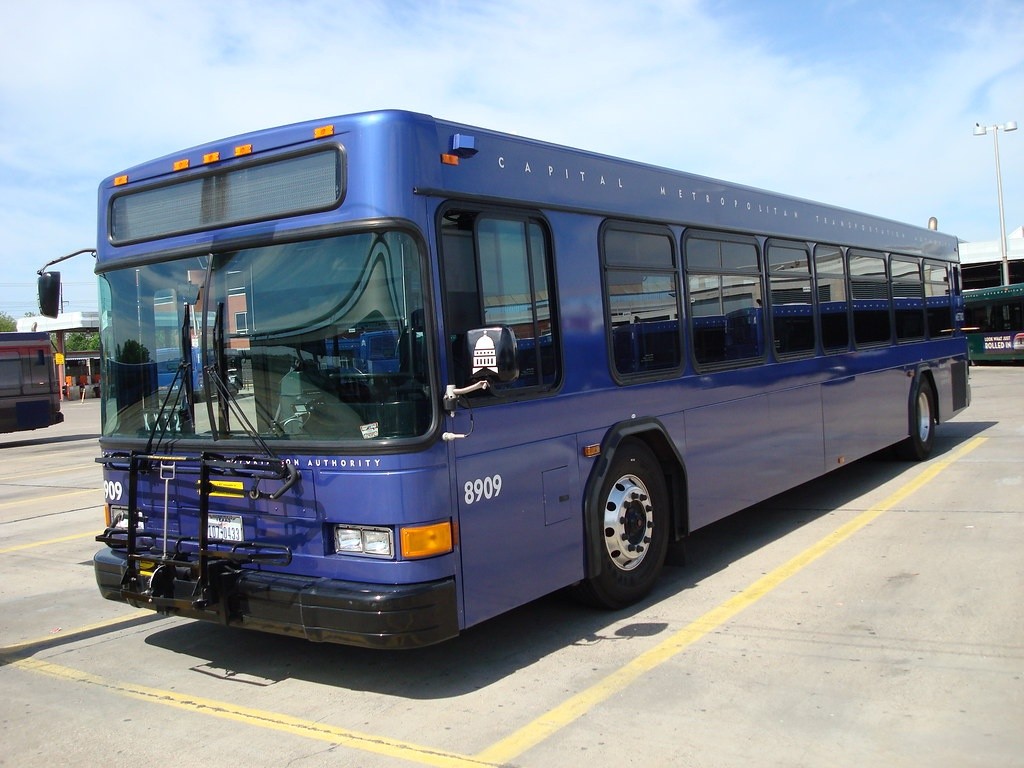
[971,119,1018,285]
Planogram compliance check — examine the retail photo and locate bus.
[36,110,971,658]
[0,333,64,438]
[961,281,1024,367]
